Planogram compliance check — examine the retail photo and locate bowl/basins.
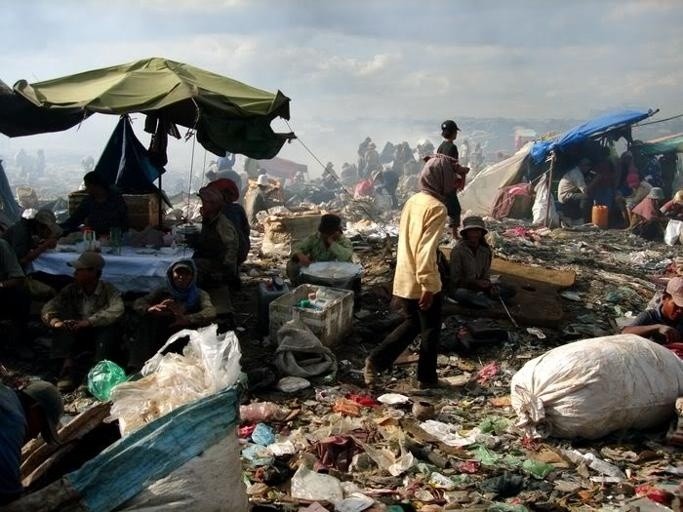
[161,247,178,254]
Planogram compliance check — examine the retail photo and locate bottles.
[170,239,177,249]
[316,287,327,297]
[299,300,311,310]
[308,293,316,303]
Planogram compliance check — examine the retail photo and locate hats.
[318,213,341,235]
[647,187,665,201]
[459,215,489,238]
[65,252,106,273]
[22,380,64,449]
[173,263,194,274]
[665,277,683,306]
[622,151,634,159]
[627,173,639,188]
[441,120,460,131]
[673,190,683,205]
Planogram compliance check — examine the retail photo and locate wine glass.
[84,230,96,250]
[109,230,124,255]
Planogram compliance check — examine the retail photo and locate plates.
[135,249,156,253]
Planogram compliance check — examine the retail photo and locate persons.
[448,215,505,316]
[296,214,362,312]
[1,240,31,359]
[436,119,464,240]
[39,250,130,393]
[181,185,240,327]
[2,380,65,508]
[56,171,130,244]
[363,155,471,390]
[125,257,217,373]
[621,276,683,344]
[557,135,683,242]
[9,208,57,266]
[210,177,252,291]
[206,134,433,224]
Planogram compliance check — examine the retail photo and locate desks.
[26,243,195,294]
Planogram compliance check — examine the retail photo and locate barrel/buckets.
[316,286,337,299]
[592,206,608,229]
[295,299,320,311]
[308,293,331,310]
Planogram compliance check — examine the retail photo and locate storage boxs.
[268,284,354,350]
[69,189,165,234]
